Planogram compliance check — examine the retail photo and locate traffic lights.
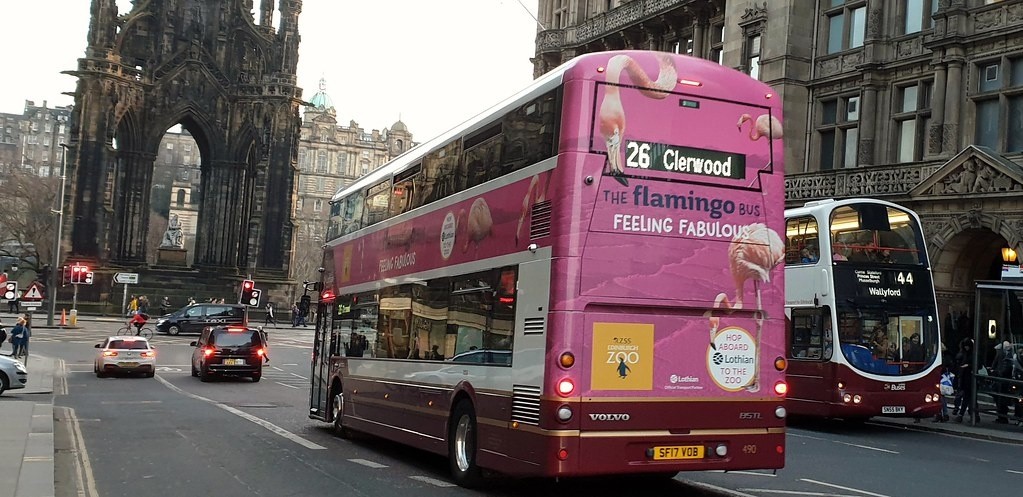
[36,264,51,286]
[71,266,93,285]
[240,279,261,308]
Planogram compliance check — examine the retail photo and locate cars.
[445,350,511,366]
[94,335,156,378]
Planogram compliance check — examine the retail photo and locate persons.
[931,337,1023,427]
[130,311,146,336]
[257,326,269,363]
[159,296,171,315]
[800,240,893,263]
[869,327,925,370]
[291,304,307,327]
[126,294,150,318]
[9,313,31,357]
[208,296,224,304]
[187,296,195,306]
[168,214,183,245]
[264,303,276,326]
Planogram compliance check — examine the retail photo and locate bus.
[784,197,944,430]
[299,50,788,490]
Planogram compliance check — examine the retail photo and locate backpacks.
[138,313,150,320]
[264,332,268,341]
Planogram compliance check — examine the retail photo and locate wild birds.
[707,221,786,393]
[456,198,494,262]
[737,113,782,189]
[515,169,552,247]
[599,52,678,188]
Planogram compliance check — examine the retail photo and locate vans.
[155,303,245,336]
[191,325,262,382]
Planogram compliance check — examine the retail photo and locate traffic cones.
[57,308,67,326]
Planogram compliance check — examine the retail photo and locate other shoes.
[15,355,24,359]
[994,417,1009,424]
[952,407,980,422]
[914,418,920,423]
[931,415,950,423]
[265,358,269,363]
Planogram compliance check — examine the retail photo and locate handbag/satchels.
[940,372,955,396]
[8,336,14,343]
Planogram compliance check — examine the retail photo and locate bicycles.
[117,321,153,342]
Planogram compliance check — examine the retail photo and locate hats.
[17,317,27,325]
[993,341,1011,356]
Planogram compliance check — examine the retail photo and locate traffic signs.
[112,273,138,284]
